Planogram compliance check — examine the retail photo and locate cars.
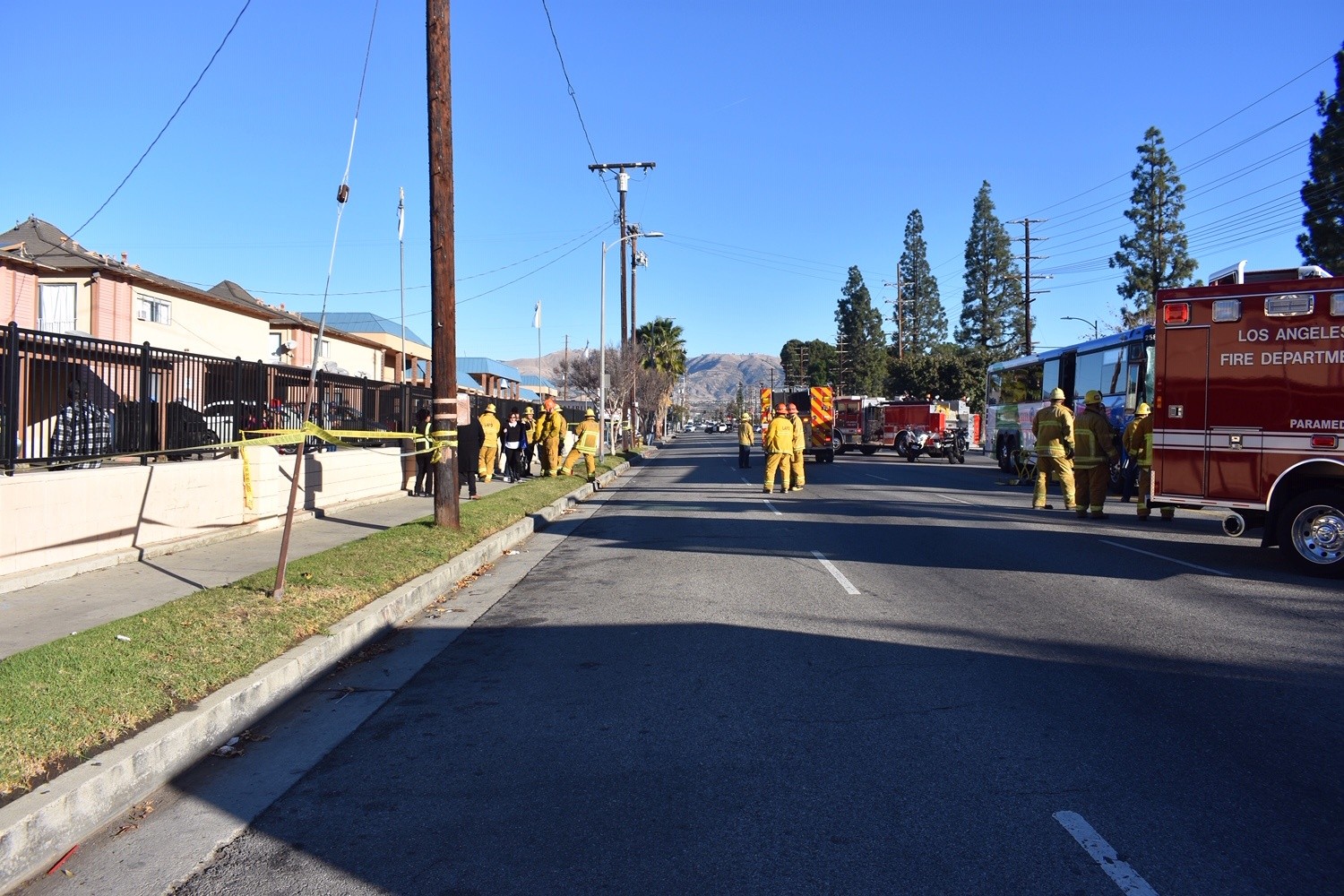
[202,400,317,454]
[685,420,733,434]
[753,425,761,433]
[329,405,392,447]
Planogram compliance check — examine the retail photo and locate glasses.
[510,417,515,418]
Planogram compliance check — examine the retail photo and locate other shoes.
[416,491,433,497]
[1121,497,1129,503]
[1161,514,1174,521]
[763,481,803,494]
[470,494,482,500]
[1033,504,1053,510]
[1077,510,1089,518]
[1066,506,1076,510]
[1139,515,1147,521]
[1090,510,1111,520]
[496,470,536,484]
[480,472,490,483]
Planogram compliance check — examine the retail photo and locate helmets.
[1135,403,1151,414]
[522,407,535,414]
[554,403,563,412]
[485,403,496,413]
[741,413,752,420]
[1085,390,1102,404]
[543,399,556,410]
[776,404,788,414]
[584,408,595,416]
[787,403,799,414]
[1050,387,1065,400]
[538,405,547,412]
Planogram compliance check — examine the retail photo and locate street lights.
[599,232,665,466]
[1060,317,1098,339]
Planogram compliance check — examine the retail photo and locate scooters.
[905,427,968,463]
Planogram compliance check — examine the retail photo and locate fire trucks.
[1149,274,1344,578]
[833,396,948,458]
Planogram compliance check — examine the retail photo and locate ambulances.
[759,385,834,462]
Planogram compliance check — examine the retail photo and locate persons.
[47,380,111,472]
[738,412,755,469]
[234,399,341,456]
[763,402,806,494]
[457,399,598,499]
[1031,387,1175,522]
[412,408,434,497]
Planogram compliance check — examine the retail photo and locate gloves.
[1065,448,1074,460]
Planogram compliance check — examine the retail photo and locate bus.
[983,324,1156,493]
